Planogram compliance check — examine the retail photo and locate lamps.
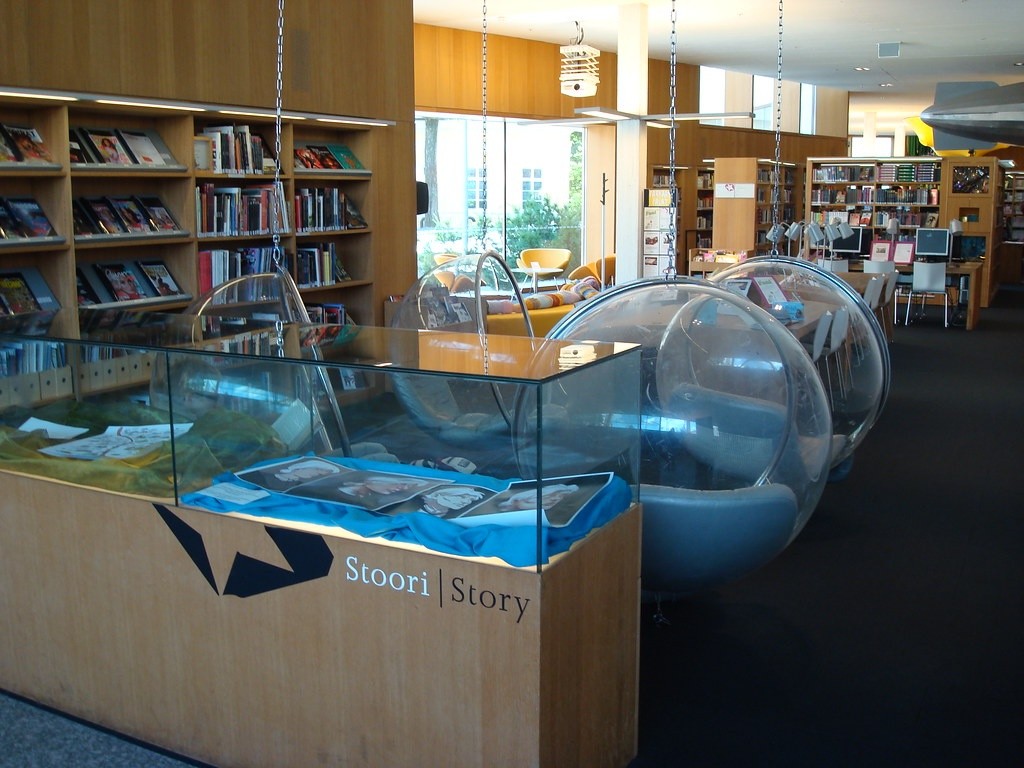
[559,21,601,98]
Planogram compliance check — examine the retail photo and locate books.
[0,122,365,332]
[655,164,1024,280]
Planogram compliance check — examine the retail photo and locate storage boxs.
[0,329,649,572]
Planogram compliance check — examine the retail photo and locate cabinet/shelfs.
[651,155,1024,249]
[0,83,397,330]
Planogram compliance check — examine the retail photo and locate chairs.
[508,273,834,606]
[862,260,899,325]
[656,254,892,483]
[817,259,849,273]
[567,255,615,288]
[516,248,573,295]
[384,250,568,450]
[904,261,949,328]
[433,268,487,298]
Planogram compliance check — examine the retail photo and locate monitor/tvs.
[952,235,986,260]
[916,227,949,256]
[816,226,874,258]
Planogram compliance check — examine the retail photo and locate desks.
[579,301,853,394]
[847,261,984,331]
[690,260,899,347]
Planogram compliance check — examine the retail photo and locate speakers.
[417,181,428,214]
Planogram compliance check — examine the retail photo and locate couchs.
[479,283,576,337]
